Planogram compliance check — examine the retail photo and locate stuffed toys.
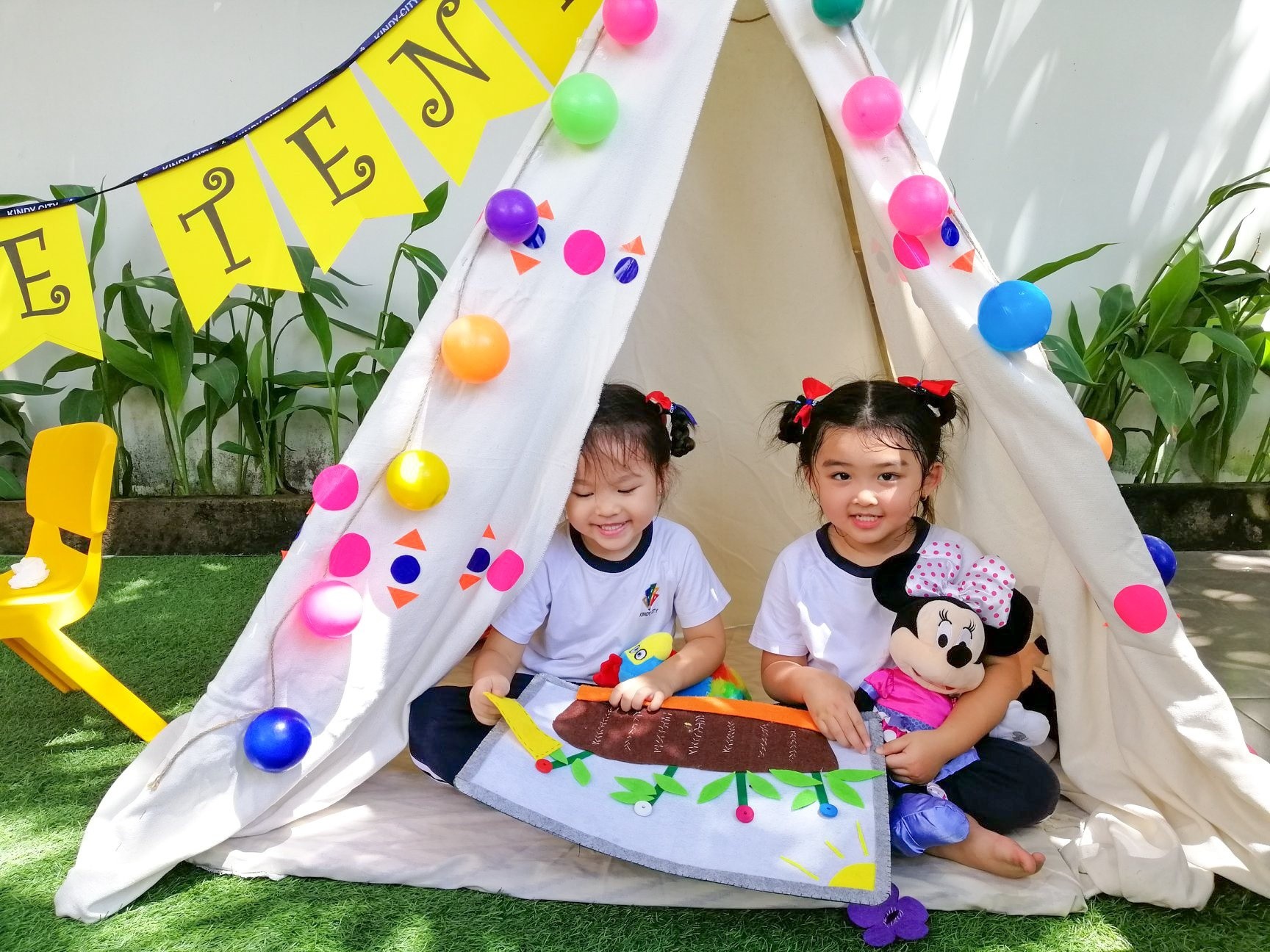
[591,630,755,705]
[852,537,1035,859]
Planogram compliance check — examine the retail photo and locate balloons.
[440,319,512,387]
[482,189,539,245]
[301,583,364,639]
[888,176,949,235]
[245,708,312,774]
[602,1,658,46]
[547,75,617,150]
[842,75,904,138]
[811,0,864,28]
[1141,534,1177,587]
[1084,418,1113,462]
[977,280,1052,353]
[384,446,451,514]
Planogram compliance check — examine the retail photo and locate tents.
[48,1,1270,915]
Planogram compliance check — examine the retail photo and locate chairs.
[0,422,167,741]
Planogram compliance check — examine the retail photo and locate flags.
[129,1,604,338]
[0,202,103,371]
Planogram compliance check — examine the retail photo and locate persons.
[747,374,1060,881]
[408,383,727,790]
